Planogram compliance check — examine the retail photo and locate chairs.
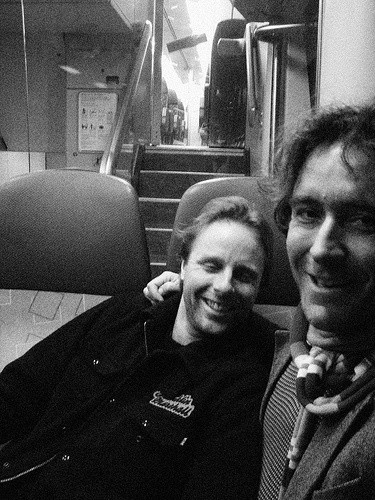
[156,177,308,333]
[159,15,248,149]
[1,167,150,378]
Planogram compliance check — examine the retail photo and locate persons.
[0,195,290,499]
[141,101,374,500]
